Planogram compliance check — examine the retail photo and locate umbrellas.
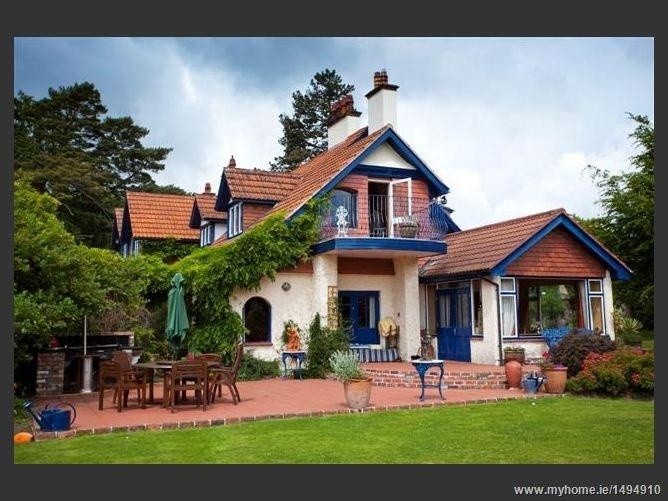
[165,274,189,362]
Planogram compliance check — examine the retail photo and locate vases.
[344,379,373,409]
[505,361,523,390]
[541,367,568,394]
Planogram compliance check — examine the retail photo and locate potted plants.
[399,221,417,238]
[505,346,525,363]
[543,353,565,368]
[276,318,303,352]
[506,347,525,364]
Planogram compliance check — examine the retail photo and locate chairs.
[99,343,243,412]
[371,210,386,237]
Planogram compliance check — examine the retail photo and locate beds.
[413,360,445,401]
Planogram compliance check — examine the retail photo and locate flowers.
[329,350,363,383]
